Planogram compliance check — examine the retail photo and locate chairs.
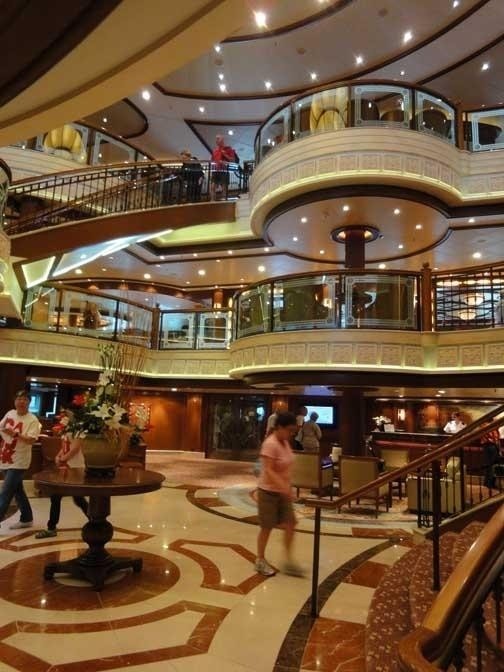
[289,444,466,521]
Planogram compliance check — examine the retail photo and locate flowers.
[52,286,156,454]
[371,415,392,426]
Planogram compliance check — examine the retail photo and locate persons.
[36,413,92,540]
[442,412,504,489]
[0,390,41,530]
[210,134,236,203]
[178,151,204,202]
[252,400,322,577]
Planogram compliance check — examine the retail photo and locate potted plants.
[218,411,255,459]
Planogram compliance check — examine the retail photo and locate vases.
[80,433,122,473]
[376,424,385,433]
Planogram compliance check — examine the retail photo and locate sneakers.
[8,520,33,530]
[279,562,308,577]
[34,529,57,539]
[253,557,276,576]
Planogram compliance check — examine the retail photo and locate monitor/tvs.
[303,405,334,426]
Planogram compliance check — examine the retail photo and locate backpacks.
[221,146,240,163]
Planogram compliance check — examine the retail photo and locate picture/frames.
[128,401,151,432]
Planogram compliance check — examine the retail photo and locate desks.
[32,464,167,594]
